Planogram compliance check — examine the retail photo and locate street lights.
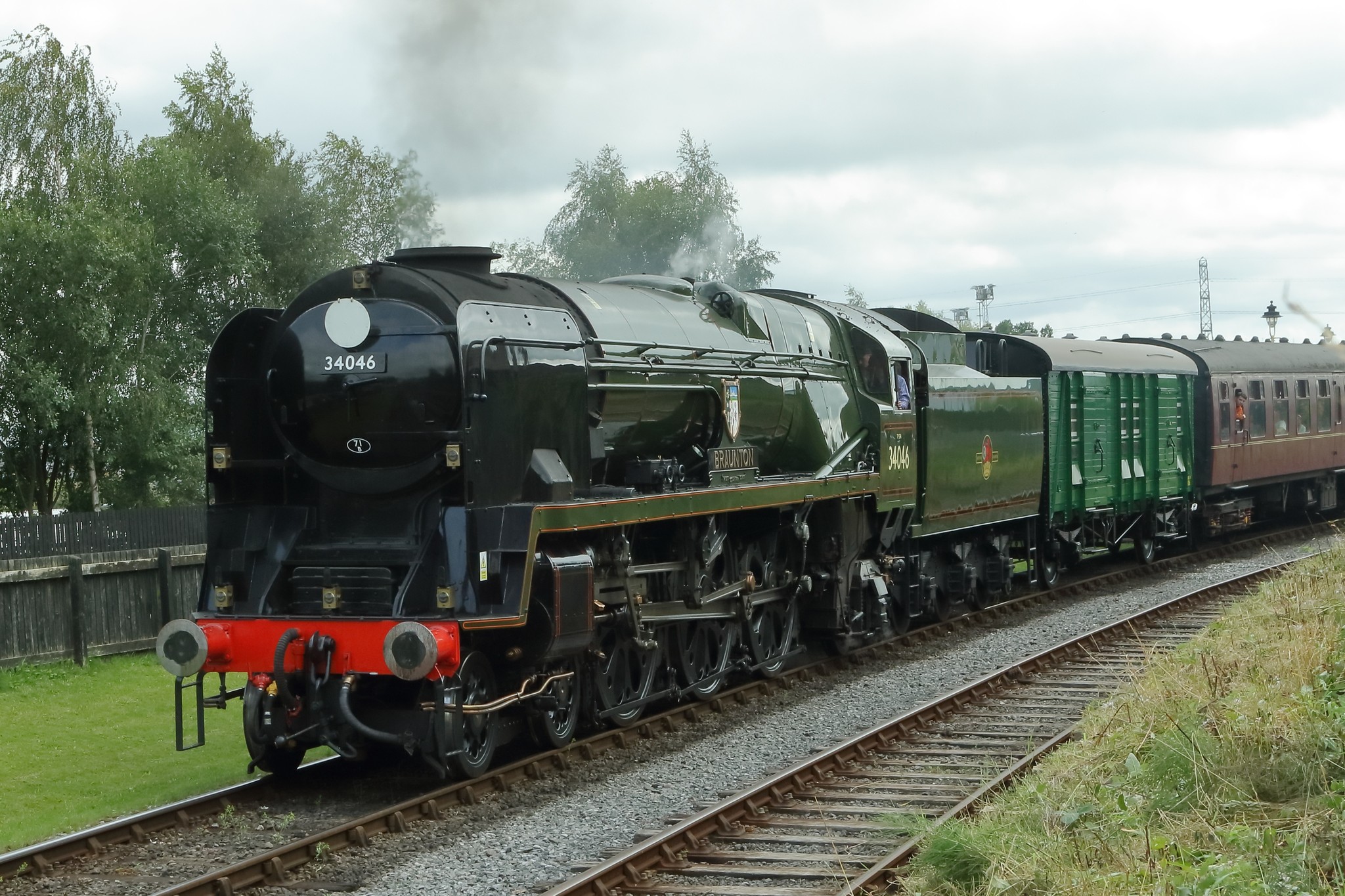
[1260,300,1283,343]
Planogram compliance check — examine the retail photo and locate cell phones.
[1243,414,1246,418]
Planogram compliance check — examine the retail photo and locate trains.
[155,246,1345,784]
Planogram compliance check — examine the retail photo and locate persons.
[1235,392,1248,420]
[1297,414,1306,433]
[858,345,911,410]
[1273,410,1288,435]
